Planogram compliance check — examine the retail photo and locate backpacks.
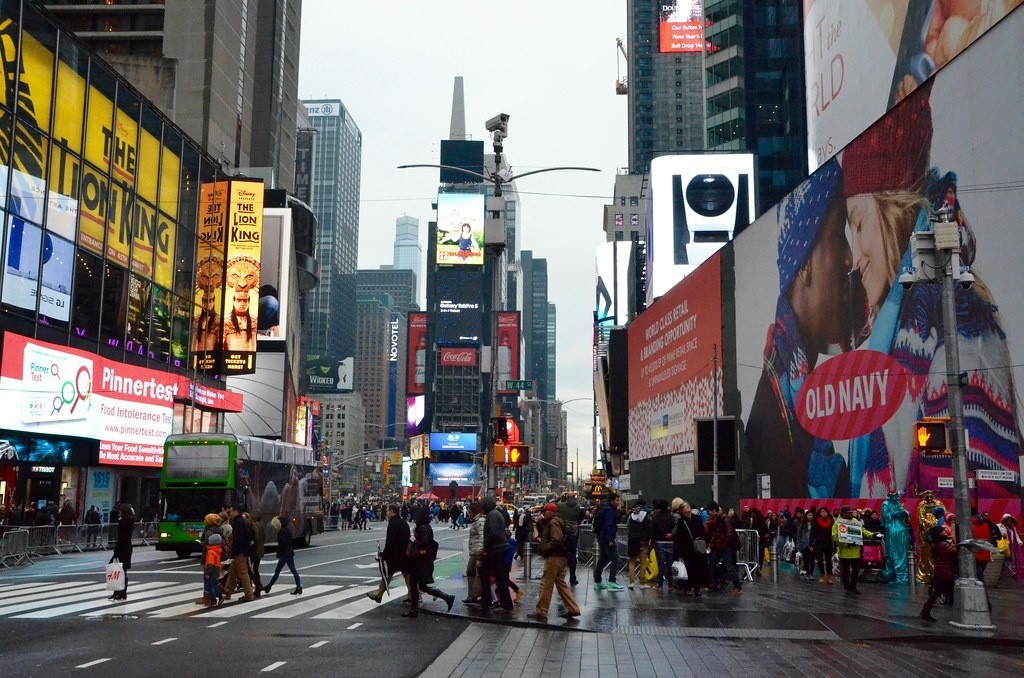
[219,540,232,562]
[593,509,603,534]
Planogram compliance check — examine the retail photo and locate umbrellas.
[418,492,439,499]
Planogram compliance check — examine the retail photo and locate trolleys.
[576,522,651,583]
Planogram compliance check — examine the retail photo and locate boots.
[108,590,118,600]
[919,602,937,622]
[113,578,128,600]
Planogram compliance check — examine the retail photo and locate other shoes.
[1010,571,1017,578]
[195,571,866,624]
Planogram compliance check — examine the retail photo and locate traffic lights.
[386,461,391,470]
[911,421,947,451]
[395,452,400,462]
[509,445,529,466]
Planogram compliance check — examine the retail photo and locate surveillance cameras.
[898,272,916,289]
[958,273,975,289]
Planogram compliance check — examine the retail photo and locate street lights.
[362,422,409,486]
[398,164,601,497]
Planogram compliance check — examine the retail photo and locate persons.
[108,501,121,544]
[0,497,74,543]
[400,511,456,618]
[365,500,388,522]
[238,464,255,515]
[254,465,323,526]
[108,504,135,599]
[594,493,884,598]
[880,489,1024,623]
[410,501,469,530]
[261,516,302,595]
[94,506,101,518]
[366,503,411,603]
[461,496,524,617]
[323,496,368,530]
[401,503,409,521]
[223,295,257,351]
[196,504,266,606]
[440,222,481,253]
[514,502,582,621]
[191,291,220,351]
[129,505,135,515]
[742,82,1024,498]
[550,492,584,584]
[84,505,102,546]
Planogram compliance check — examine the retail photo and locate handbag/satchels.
[106,558,125,591]
[780,537,795,563]
[645,538,659,582]
[546,517,571,550]
[762,546,771,562]
[672,560,688,581]
[406,525,429,559]
[693,537,706,554]
[722,516,741,552]
[996,537,1011,558]
[794,551,806,575]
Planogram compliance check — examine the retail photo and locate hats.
[517,507,524,515]
[775,156,843,294]
[208,534,222,546]
[842,505,853,513]
[543,503,558,513]
[205,514,222,526]
[842,74,935,198]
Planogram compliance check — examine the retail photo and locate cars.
[455,493,546,525]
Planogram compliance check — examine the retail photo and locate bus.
[156,431,325,556]
